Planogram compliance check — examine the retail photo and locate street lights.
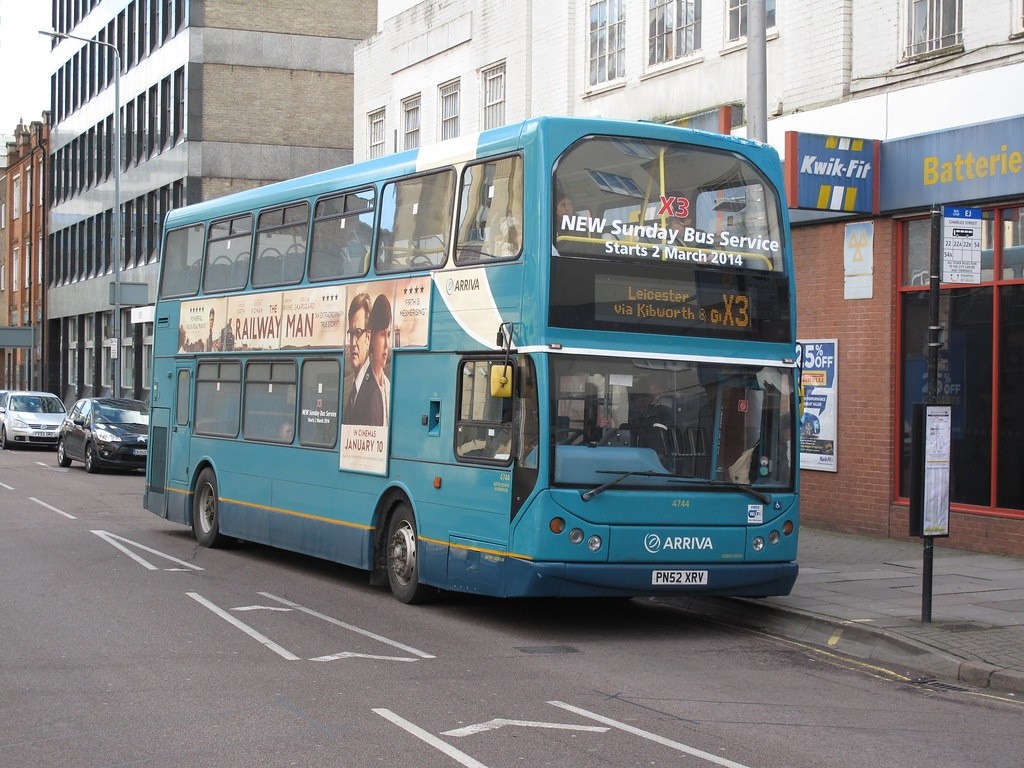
[36,28,123,393]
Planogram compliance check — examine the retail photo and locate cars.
[58,396,153,473]
[0,389,68,450]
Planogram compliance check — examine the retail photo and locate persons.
[556,193,589,237]
[638,210,696,248]
[748,413,803,485]
[492,409,538,454]
[344,292,383,426]
[494,216,559,257]
[279,424,293,439]
[371,294,392,424]
[600,380,680,473]
[204,308,215,352]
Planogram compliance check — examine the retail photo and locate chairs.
[188,240,433,289]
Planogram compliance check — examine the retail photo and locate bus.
[144,114,807,607]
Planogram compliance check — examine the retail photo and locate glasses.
[346,328,367,341]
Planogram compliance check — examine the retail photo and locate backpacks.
[729,440,764,484]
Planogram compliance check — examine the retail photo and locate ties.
[344,382,357,424]
